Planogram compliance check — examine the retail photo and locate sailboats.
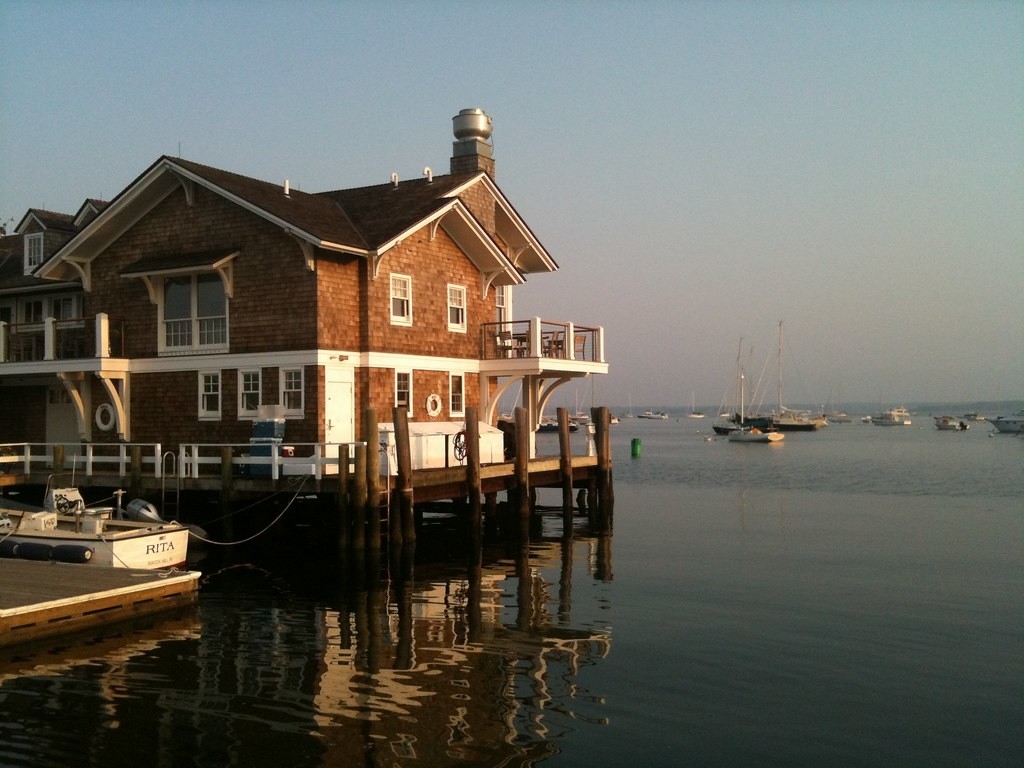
[709,318,874,443]
[684,390,706,419]
[502,375,667,433]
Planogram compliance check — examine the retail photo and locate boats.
[872,403,914,427]
[935,416,971,431]
[0,472,190,571]
[965,413,986,421]
[985,408,1024,434]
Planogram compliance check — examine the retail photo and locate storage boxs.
[257,405,284,419]
[283,464,315,475]
[249,419,285,478]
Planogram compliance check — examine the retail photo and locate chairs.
[499,331,586,359]
[8,333,28,363]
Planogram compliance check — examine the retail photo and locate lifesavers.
[426,395,441,416]
[95,403,113,432]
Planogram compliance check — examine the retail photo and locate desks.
[510,336,549,358]
[19,334,44,361]
